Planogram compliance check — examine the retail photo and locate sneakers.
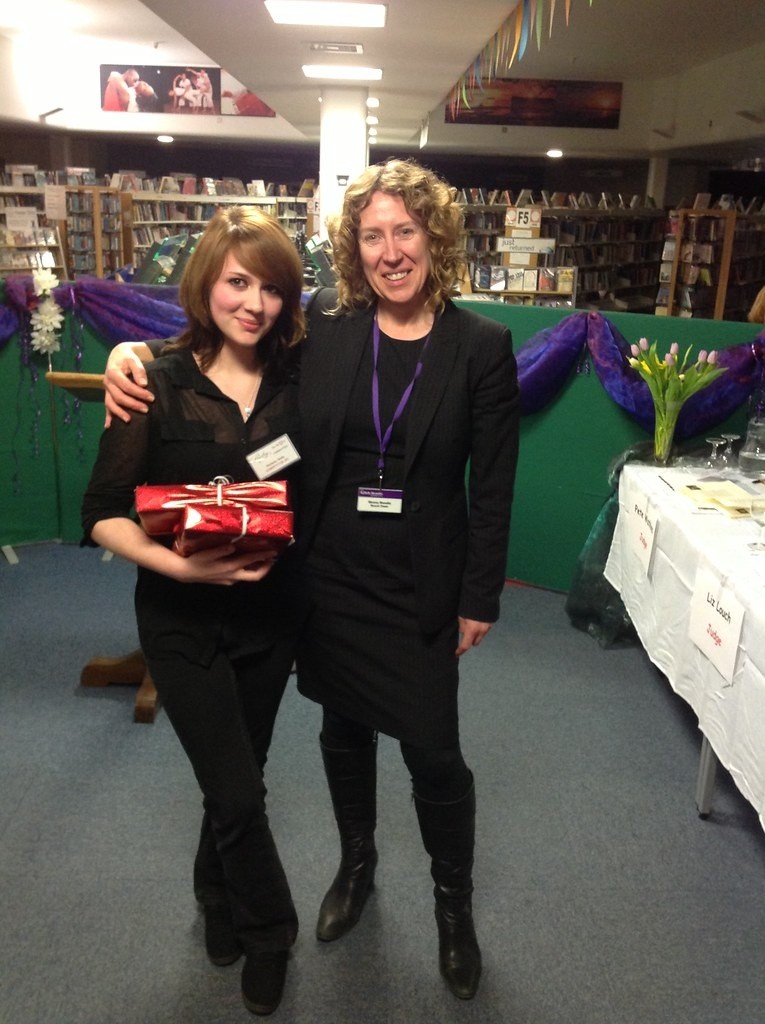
[204,902,244,966]
[241,950,289,1016]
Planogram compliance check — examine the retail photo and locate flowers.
[627,336,719,398]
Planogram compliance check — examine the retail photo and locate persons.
[79,202,315,1015]
[102,66,249,115]
[104,161,526,999]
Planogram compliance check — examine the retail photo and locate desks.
[625,464,764,820]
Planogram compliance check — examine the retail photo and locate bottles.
[738,417,765,478]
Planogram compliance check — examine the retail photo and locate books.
[449,186,765,325]
[0,162,319,285]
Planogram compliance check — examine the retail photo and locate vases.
[652,399,683,468]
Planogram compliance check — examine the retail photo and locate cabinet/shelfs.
[0,182,763,325]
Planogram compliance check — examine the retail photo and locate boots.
[409,766,482,1002]
[314,728,381,945]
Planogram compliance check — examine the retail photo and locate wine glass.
[718,433,742,476]
[705,437,727,478]
